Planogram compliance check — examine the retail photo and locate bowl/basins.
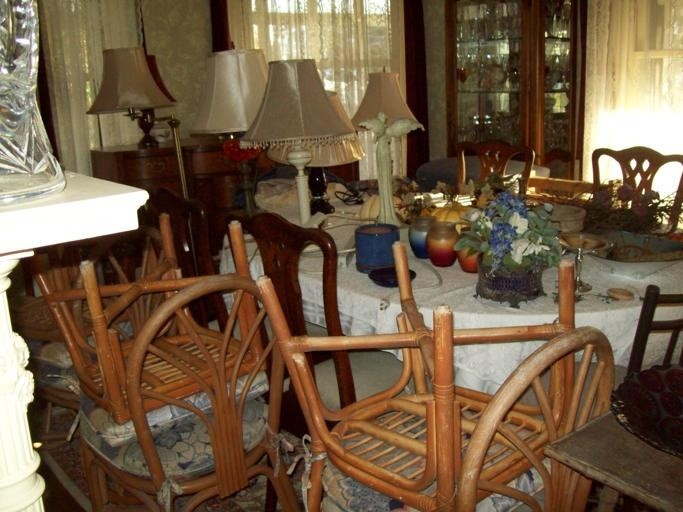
[545,205,586,234]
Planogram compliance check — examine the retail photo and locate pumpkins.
[431,202,474,235]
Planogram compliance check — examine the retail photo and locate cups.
[456,3,572,147]
[408,216,479,273]
[353,225,402,272]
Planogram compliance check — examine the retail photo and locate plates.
[366,268,418,286]
[609,361,682,461]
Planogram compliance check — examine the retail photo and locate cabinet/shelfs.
[443,0,588,181]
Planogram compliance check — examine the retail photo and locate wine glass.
[558,230,614,295]
